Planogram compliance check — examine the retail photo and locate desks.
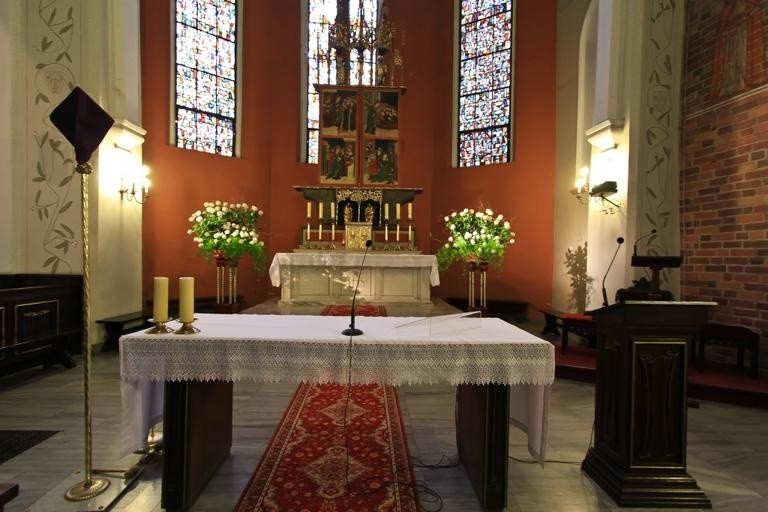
[120,313,554,510]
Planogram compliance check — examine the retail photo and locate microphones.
[634,229,656,256]
[342,240,373,336]
[602,237,624,306]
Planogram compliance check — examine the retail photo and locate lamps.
[590,181,621,209]
[572,185,588,204]
[117,163,152,203]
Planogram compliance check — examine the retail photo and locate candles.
[385,204,388,220]
[179,277,194,321]
[152,277,169,319]
[331,202,335,219]
[385,226,389,241]
[409,226,412,241]
[396,203,400,220]
[332,225,335,240]
[397,225,400,241]
[318,201,323,219]
[307,224,311,240]
[318,225,322,240]
[307,202,312,219]
[407,203,412,219]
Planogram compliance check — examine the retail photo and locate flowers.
[187,200,264,254]
[436,207,515,266]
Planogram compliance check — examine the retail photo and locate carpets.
[1,430,61,469]
[233,304,421,509]
